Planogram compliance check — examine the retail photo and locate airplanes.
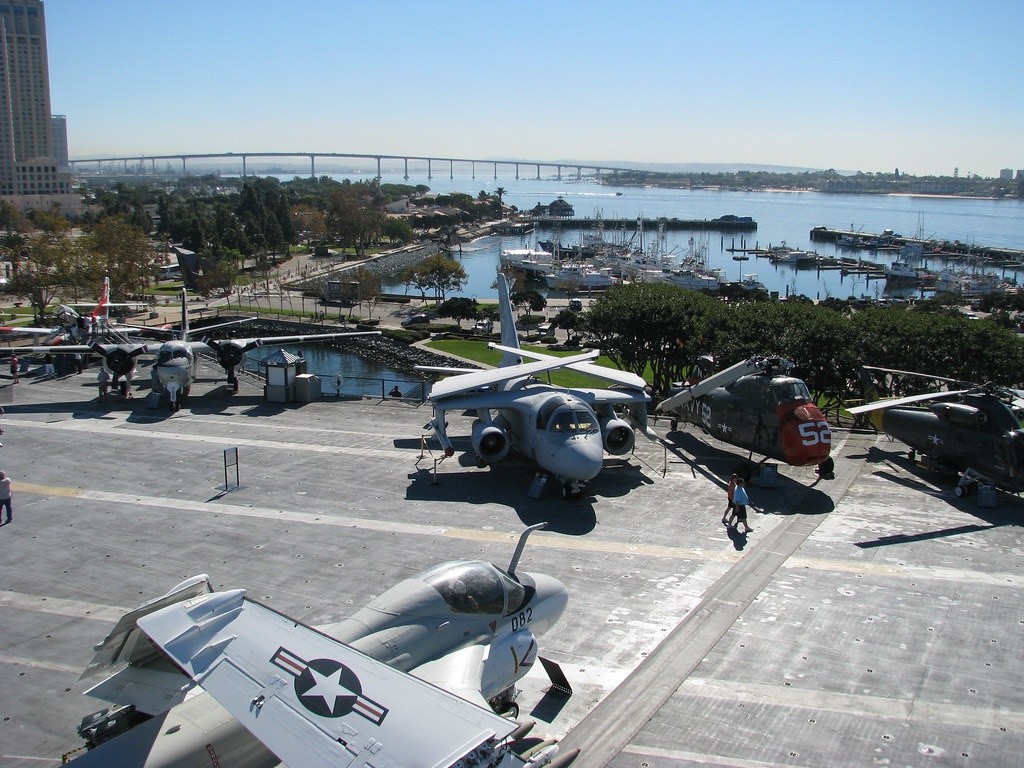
[660,353,835,486]
[413,273,658,502]
[0,274,382,411]
[59,520,584,768]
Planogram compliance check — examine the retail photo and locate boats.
[779,251,813,262]
[836,211,1007,297]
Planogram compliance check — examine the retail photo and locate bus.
[158,264,182,281]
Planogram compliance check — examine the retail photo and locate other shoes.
[722,519,729,523]
[727,524,732,529]
[745,527,753,532]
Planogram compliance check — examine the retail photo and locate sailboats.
[496,206,722,291]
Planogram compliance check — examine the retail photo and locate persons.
[389,386,402,397]
[722,474,754,532]
[0,471,12,524]
[75,354,82,374]
[151,365,161,393]
[44,353,55,376]
[314,310,325,322]
[97,368,110,400]
[10,354,19,384]
[70,312,97,344]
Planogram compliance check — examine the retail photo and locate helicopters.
[842,364,1024,499]
